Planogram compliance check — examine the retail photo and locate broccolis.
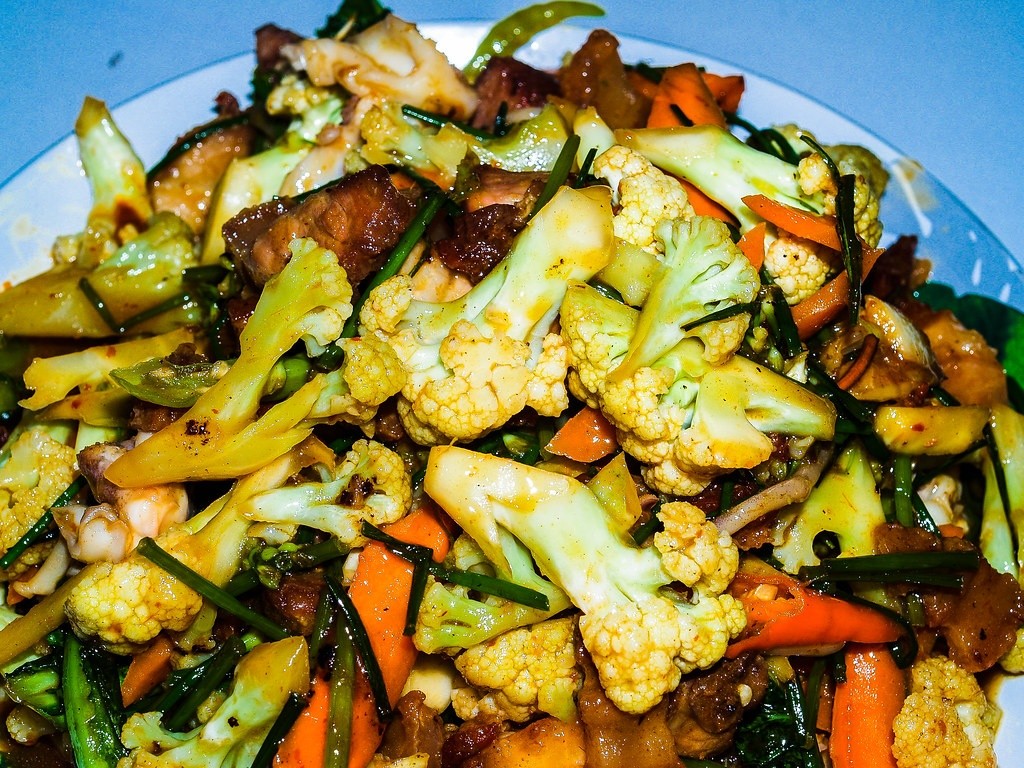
[0,16,1024,768]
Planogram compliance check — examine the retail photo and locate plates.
[0,22,1024,768]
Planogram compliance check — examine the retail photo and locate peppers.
[458,1,607,78]
